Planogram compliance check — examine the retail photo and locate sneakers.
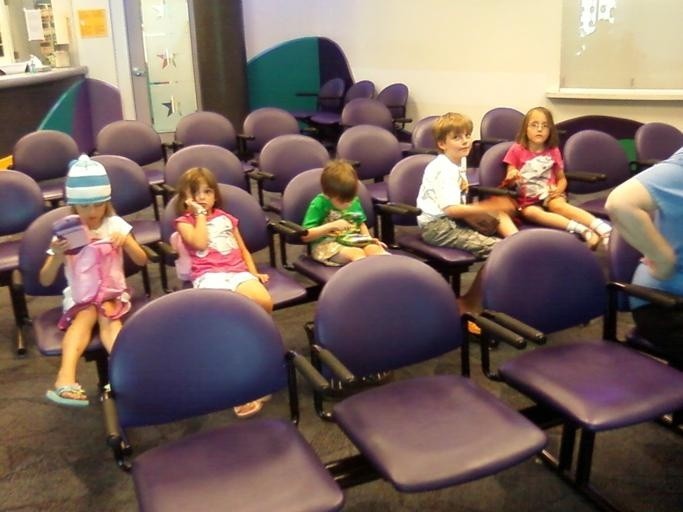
[580,228,598,249]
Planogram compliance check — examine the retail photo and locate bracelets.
[192,209,208,216]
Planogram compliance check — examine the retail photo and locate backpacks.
[56,239,131,330]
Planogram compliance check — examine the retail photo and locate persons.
[502,107,613,251]
[299,159,391,266]
[38,152,148,407]
[170,167,273,417]
[417,112,520,315]
[604,147,683,371]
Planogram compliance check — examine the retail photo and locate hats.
[65,155,111,204]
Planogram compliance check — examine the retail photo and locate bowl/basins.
[0,62,27,74]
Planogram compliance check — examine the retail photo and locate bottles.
[55,51,65,68]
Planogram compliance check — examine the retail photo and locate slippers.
[46,384,89,407]
